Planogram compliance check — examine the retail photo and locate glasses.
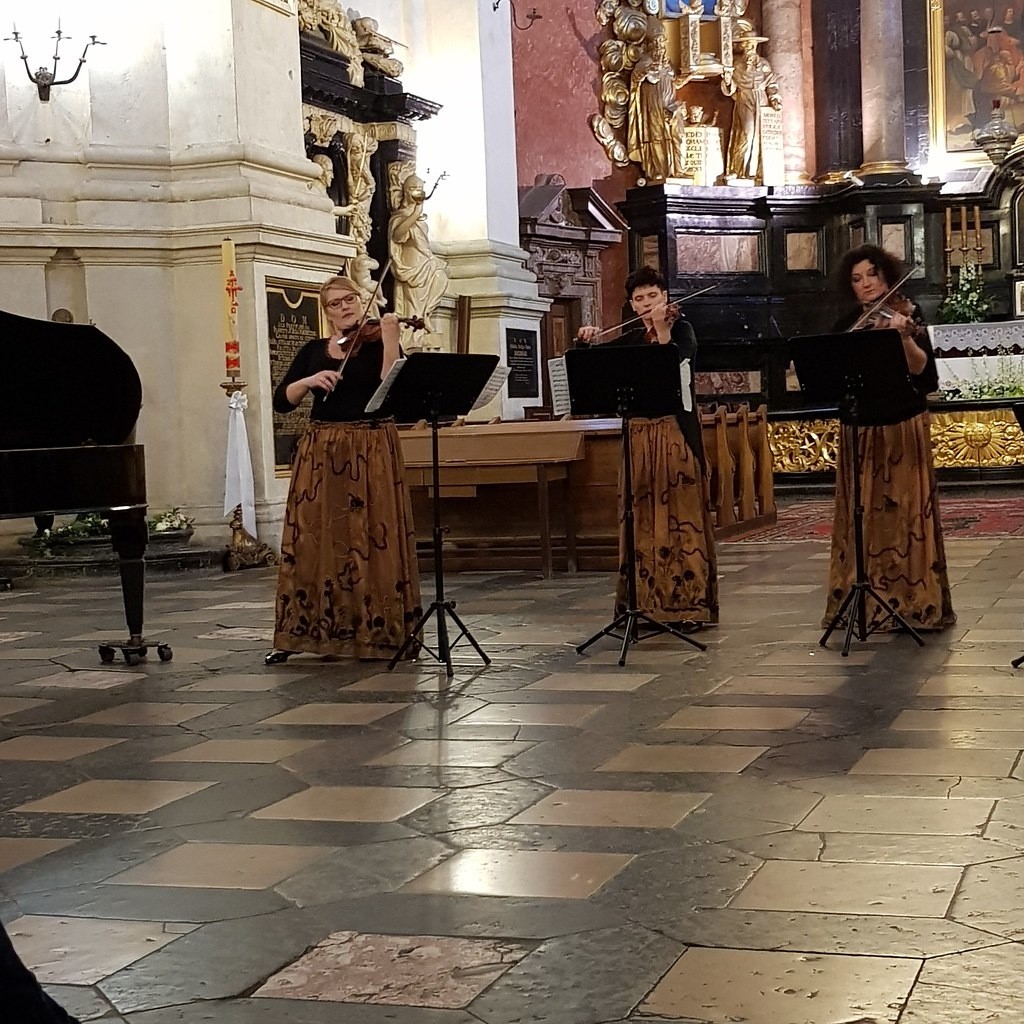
[324,293,359,309]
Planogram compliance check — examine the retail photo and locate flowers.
[933,259,1002,324]
[29,505,196,558]
[938,341,1024,400]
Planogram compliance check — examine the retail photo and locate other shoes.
[681,620,702,633]
[264,649,302,664]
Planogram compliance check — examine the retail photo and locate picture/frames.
[927,0,1024,171]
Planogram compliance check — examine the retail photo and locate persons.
[574,266,718,632]
[389,174,449,333]
[262,276,425,665]
[312,154,357,217]
[720,29,782,180]
[820,244,956,633]
[625,15,693,178]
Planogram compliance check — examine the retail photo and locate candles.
[960,206,968,247]
[220,234,241,378]
[974,206,983,247]
[946,207,951,248]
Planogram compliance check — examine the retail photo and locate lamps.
[969,100,1024,182]
[3,16,107,101]
[492,0,542,30]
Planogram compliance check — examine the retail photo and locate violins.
[858,293,928,338]
[336,314,426,354]
[645,303,682,344]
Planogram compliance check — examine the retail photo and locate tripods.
[788,328,926,658]
[565,342,708,666]
[374,353,500,677]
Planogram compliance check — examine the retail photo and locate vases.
[19,528,195,557]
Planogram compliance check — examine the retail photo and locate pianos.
[0,310,178,666]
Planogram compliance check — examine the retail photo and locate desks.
[397,458,588,575]
